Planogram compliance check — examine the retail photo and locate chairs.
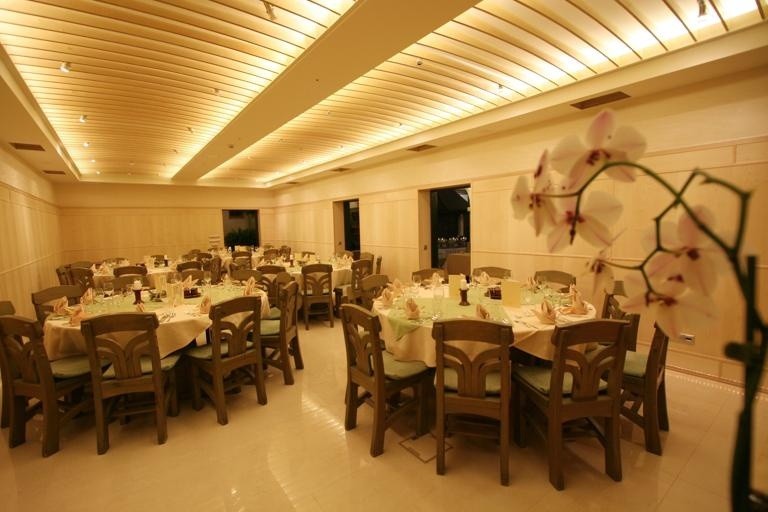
[344,273,419,409]
[588,291,640,354]
[514,318,632,491]
[472,266,511,281]
[338,303,435,456]
[412,267,450,284]
[431,320,513,487]
[533,271,577,290]
[585,321,669,456]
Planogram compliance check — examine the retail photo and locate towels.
[567,284,581,303]
[404,298,419,320]
[560,292,588,315]
[531,300,556,325]
[476,270,490,285]
[475,304,490,320]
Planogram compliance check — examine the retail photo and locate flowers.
[511,111,768,512]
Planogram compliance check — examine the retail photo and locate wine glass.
[80,273,233,318]
[413,274,549,320]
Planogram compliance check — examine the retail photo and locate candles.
[461,279,467,291]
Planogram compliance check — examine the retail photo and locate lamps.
[60,60,72,74]
